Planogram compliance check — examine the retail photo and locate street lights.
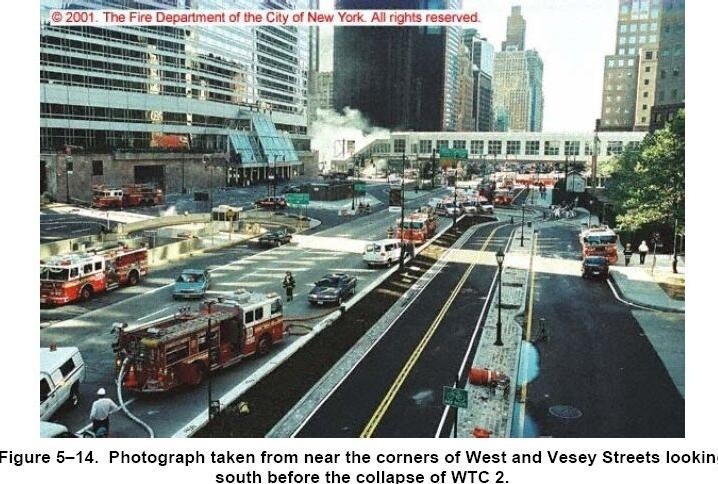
[587,196,594,228]
[493,246,505,347]
[267,167,277,231]
[520,198,527,247]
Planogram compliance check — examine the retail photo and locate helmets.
[97,388,106,395]
[96,427,108,436]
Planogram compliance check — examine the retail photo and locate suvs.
[581,255,610,281]
[253,195,287,209]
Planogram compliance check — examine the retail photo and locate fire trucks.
[579,222,619,265]
[108,287,286,397]
[90,182,164,209]
[40,244,148,307]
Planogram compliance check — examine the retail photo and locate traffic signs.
[440,147,453,158]
[453,148,468,158]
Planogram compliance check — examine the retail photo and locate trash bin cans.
[528,221,531,227]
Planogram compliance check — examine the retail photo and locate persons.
[638,241,649,267]
[89,386,120,438]
[282,271,296,303]
[549,201,577,219]
[622,243,633,267]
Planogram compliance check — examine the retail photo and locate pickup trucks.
[40,344,87,422]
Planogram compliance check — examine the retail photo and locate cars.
[307,271,358,306]
[282,185,300,193]
[258,230,293,249]
[172,267,211,299]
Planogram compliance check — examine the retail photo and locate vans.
[39,420,97,437]
[361,170,513,270]
